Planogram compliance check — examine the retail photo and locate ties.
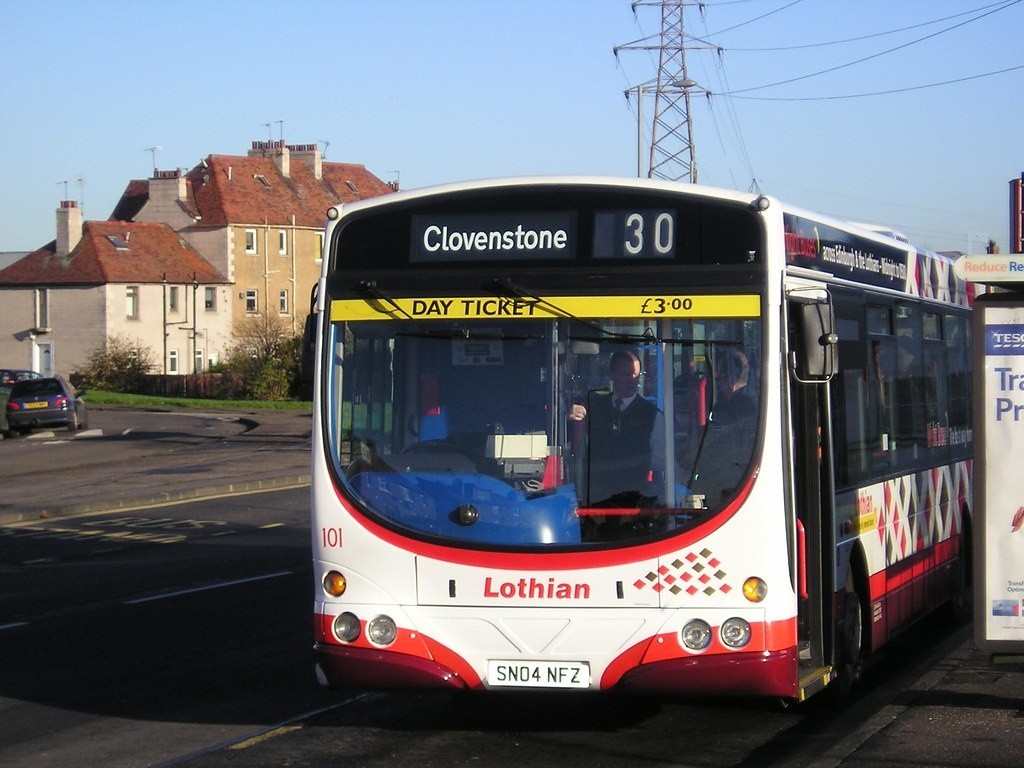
[613,398,623,432]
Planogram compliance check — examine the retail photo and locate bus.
[298,177,985,712]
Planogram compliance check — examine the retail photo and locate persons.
[691,351,759,506]
[565,349,665,503]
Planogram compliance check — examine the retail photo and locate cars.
[0,368,45,390]
[5,375,87,434]
